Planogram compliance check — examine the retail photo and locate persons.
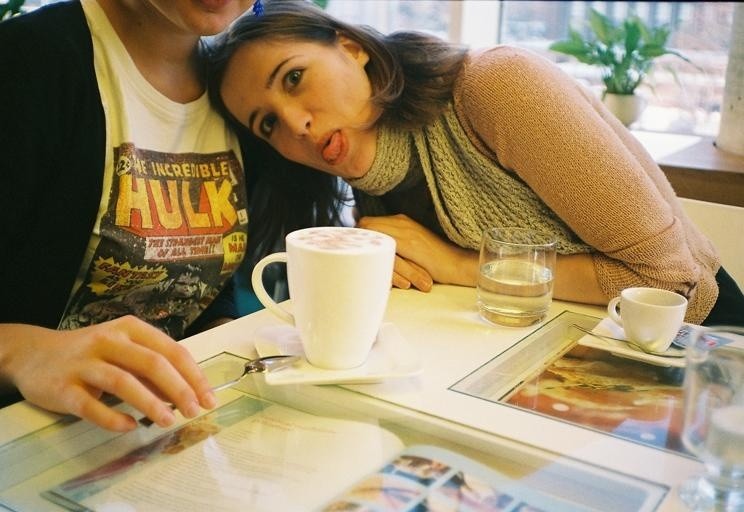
[0,0,342,434]
[202,0,744,331]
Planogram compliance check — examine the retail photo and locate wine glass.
[679,326,744,512]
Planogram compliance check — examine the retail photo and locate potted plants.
[549,5,705,125]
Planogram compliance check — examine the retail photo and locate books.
[39,394,595,512]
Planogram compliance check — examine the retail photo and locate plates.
[576,316,711,373]
[247,323,423,386]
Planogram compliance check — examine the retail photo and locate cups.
[476,228,560,326]
[605,287,688,356]
[253,226,397,371]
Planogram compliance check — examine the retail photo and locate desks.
[2,283,744,512]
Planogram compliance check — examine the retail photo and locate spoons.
[570,323,686,358]
[137,356,299,426]
